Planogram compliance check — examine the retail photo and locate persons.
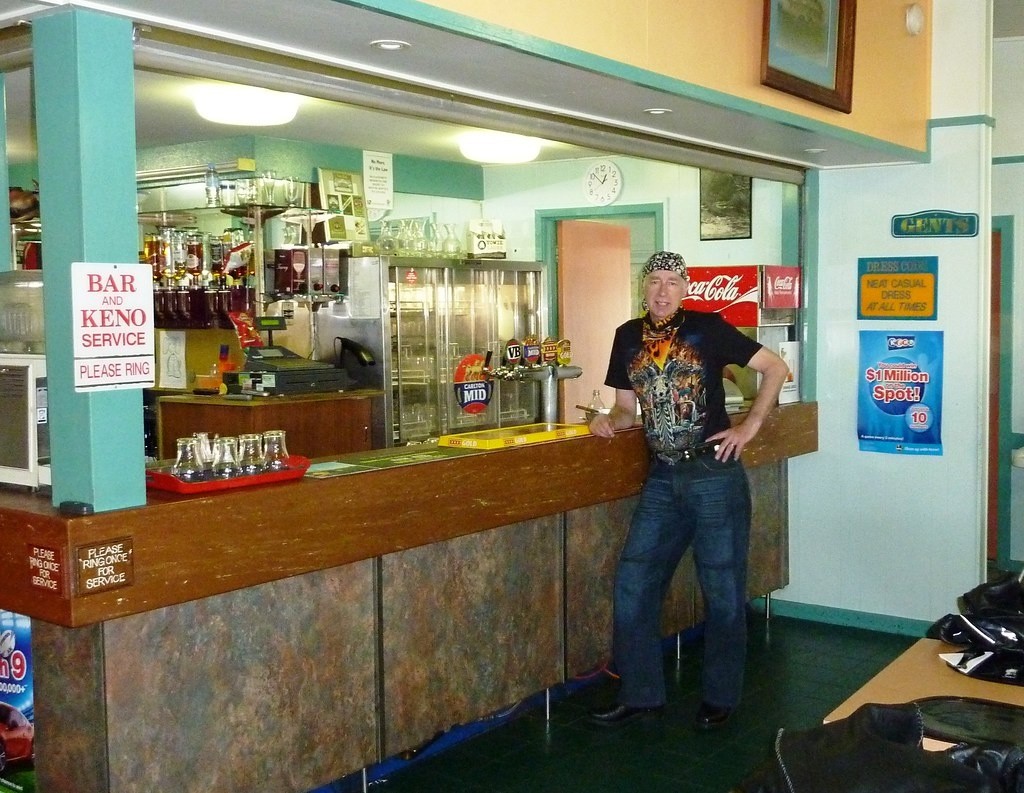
[586,249,790,728]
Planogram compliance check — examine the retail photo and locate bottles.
[205,163,221,207]
[379,211,462,254]
[585,389,605,422]
[138,222,245,286]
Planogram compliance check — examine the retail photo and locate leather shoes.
[586,700,665,726]
[694,701,732,727]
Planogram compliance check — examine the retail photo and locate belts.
[649,441,724,466]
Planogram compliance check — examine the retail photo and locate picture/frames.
[760,0,857,114]
[700,166,752,242]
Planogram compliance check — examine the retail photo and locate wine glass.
[262,170,280,206]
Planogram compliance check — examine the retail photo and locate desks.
[822,637,1023,751]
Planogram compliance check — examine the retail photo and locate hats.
[642,251,687,281]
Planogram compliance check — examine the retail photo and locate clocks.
[581,160,624,207]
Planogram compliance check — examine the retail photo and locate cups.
[234,178,258,205]
[395,355,493,428]
[283,176,299,207]
[173,429,290,484]
[154,291,232,315]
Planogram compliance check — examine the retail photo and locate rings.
[728,442,735,447]
